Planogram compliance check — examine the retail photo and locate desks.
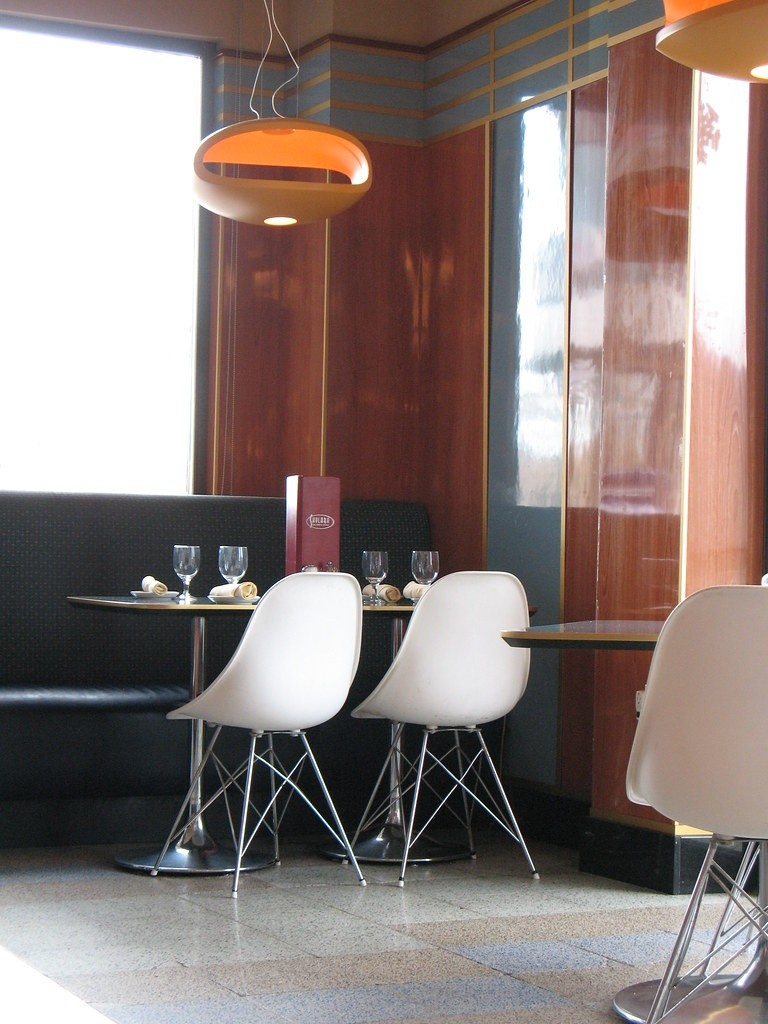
[502,615,768,1024]
[70,595,478,874]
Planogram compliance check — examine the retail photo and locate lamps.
[189,0,374,227]
[650,0,768,86]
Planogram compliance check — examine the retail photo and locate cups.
[411,551,439,585]
[219,546,248,584]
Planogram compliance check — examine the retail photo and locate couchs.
[0,489,480,843]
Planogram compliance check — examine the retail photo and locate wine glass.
[172,544,200,601]
[361,550,388,603]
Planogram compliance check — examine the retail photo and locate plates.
[130,590,180,598]
[207,595,260,604]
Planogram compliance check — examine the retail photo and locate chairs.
[145,571,367,899]
[346,569,537,885]
[618,584,768,1024]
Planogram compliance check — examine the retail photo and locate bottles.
[322,561,341,572]
[302,563,318,572]
